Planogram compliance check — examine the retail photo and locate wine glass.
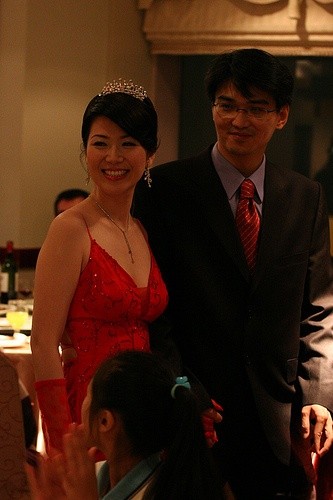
[6,305,28,343]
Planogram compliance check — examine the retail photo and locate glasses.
[210,99,278,120]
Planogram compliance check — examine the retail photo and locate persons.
[130,49,333,500]
[23,349,224,500]
[31,77,168,470]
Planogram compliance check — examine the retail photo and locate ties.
[235,179,261,277]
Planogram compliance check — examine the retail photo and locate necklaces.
[87,194,135,264]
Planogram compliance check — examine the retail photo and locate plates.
[0,339,25,348]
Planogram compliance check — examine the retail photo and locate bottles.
[0,240,21,304]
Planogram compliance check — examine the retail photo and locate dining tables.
[0,298,63,448]
